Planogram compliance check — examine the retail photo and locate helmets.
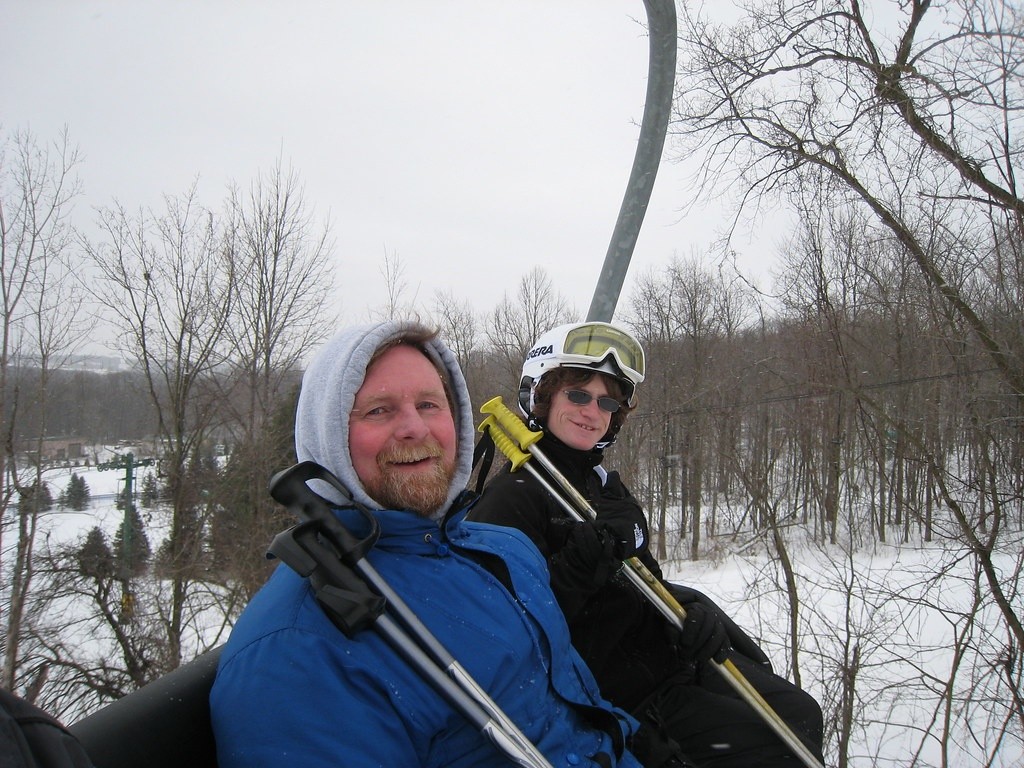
[519,323,637,423]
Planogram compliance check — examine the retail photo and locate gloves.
[679,601,733,663]
[571,471,649,573]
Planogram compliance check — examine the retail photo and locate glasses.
[556,322,645,381]
[555,389,621,412]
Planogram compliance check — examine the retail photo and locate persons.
[209,322,826,768]
